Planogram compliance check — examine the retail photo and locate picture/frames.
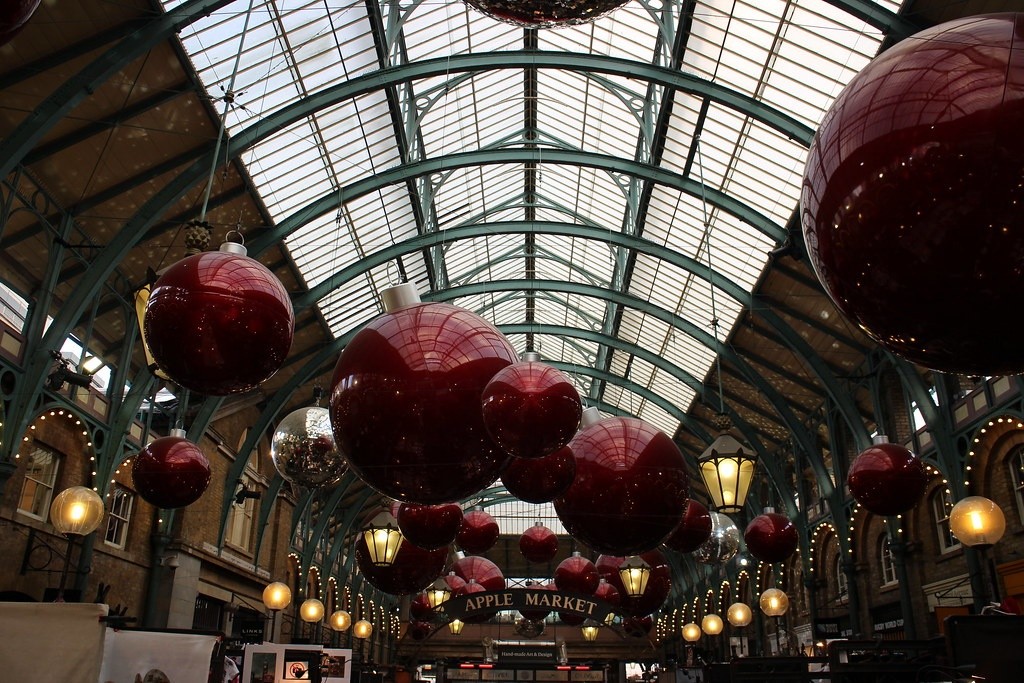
[242,643,352,683]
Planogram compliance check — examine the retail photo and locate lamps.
[760,588,789,651]
[701,614,723,649]
[48,488,105,601]
[361,508,405,567]
[426,573,452,614]
[580,617,603,642]
[300,599,325,643]
[131,0,256,381]
[262,581,292,643]
[727,603,752,655]
[331,610,351,647]
[617,555,651,598]
[449,619,464,635]
[355,620,373,655]
[949,495,1005,576]
[692,133,759,513]
[682,623,701,658]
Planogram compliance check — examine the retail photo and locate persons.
[780,633,790,656]
